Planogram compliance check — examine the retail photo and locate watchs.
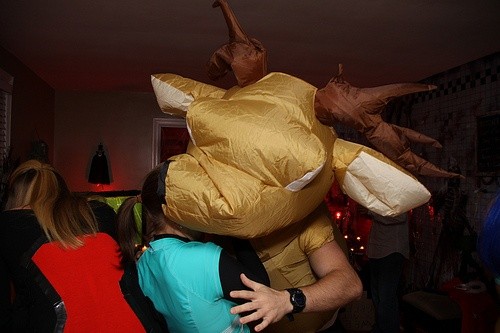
[283,287,307,323]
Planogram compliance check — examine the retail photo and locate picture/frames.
[151,118,189,169]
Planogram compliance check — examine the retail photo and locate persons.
[151,0,474,333]
[116,164,267,333]
[357,202,410,333]
[2,158,171,333]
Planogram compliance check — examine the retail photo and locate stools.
[401,292,461,333]
